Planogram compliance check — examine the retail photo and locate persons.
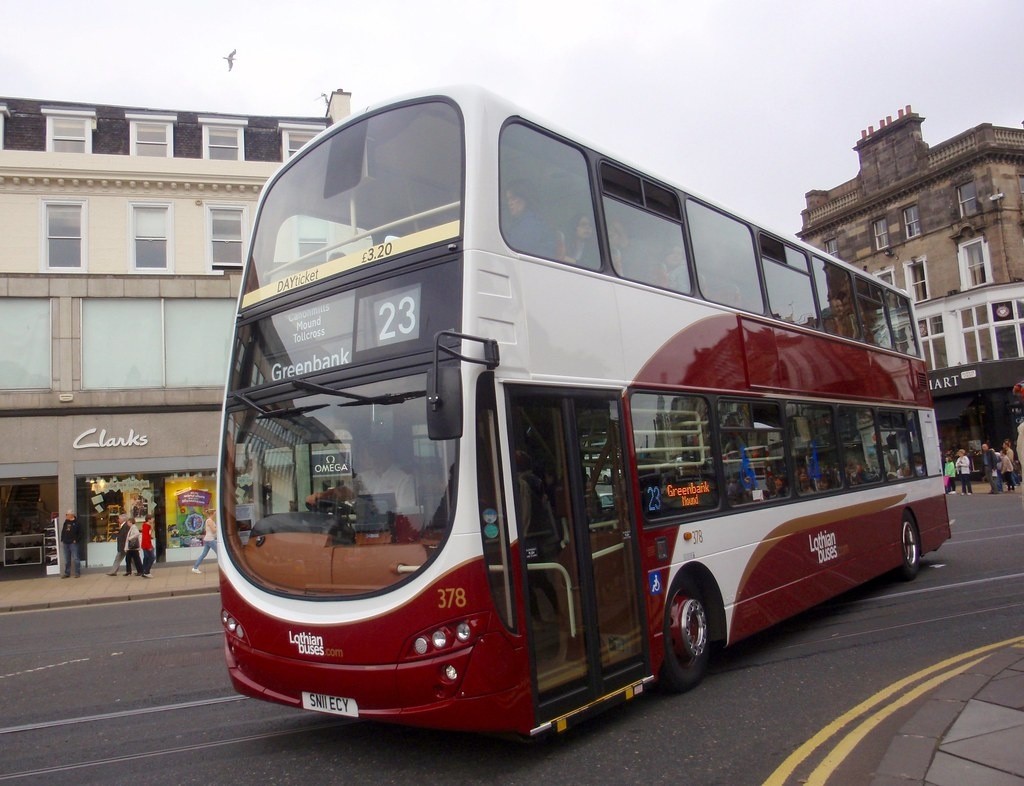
[430,177,926,540]
[123,518,143,576]
[982,443,999,495]
[944,456,957,495]
[106,514,138,576]
[60,509,81,578]
[1000,450,1015,492]
[989,446,1003,493]
[191,509,217,574]
[305,443,418,517]
[141,515,156,578]
[955,449,973,495]
[1003,440,1016,486]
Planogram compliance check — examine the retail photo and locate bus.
[219,88,953,736]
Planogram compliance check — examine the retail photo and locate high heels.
[136,573,143,576]
[123,572,132,576]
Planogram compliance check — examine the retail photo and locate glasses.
[66,514,69,516]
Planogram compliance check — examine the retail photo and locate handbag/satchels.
[129,538,140,548]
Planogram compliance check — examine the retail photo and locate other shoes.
[106,572,117,576]
[61,575,80,579]
[142,574,155,578]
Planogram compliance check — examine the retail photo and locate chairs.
[532,223,894,347]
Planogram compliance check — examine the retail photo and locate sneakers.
[192,568,202,574]
